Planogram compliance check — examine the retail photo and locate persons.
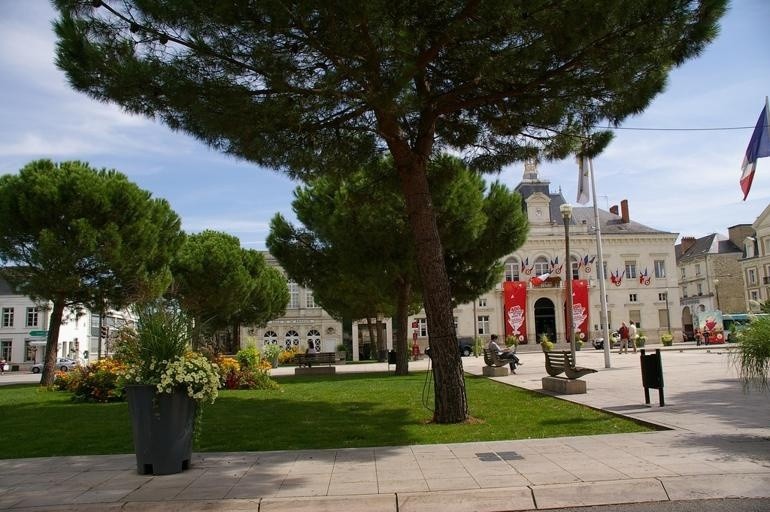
[701,326,712,346]
[693,328,703,346]
[626,319,637,352]
[682,330,688,343]
[488,334,525,375]
[302,341,319,369]
[733,318,742,326]
[616,321,629,355]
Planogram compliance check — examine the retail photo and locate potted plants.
[505,336,519,354]
[473,336,482,355]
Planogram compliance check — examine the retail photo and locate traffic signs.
[29,330,48,337]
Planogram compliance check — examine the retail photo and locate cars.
[31,357,77,373]
[593,331,640,349]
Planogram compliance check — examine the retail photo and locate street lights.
[713,279,722,311]
[559,202,577,367]
[664,287,672,335]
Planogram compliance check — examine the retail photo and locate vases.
[124,383,194,475]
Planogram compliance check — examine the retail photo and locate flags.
[739,95,770,202]
[576,125,590,206]
[519,253,655,288]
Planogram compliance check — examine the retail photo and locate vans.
[424,336,473,357]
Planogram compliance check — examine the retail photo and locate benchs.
[295,352,335,368]
[483,349,514,367]
[544,350,598,379]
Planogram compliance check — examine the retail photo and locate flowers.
[537,328,673,353]
[120,301,221,441]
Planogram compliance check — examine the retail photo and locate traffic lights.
[101,325,109,338]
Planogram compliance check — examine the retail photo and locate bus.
[722,313,770,343]
